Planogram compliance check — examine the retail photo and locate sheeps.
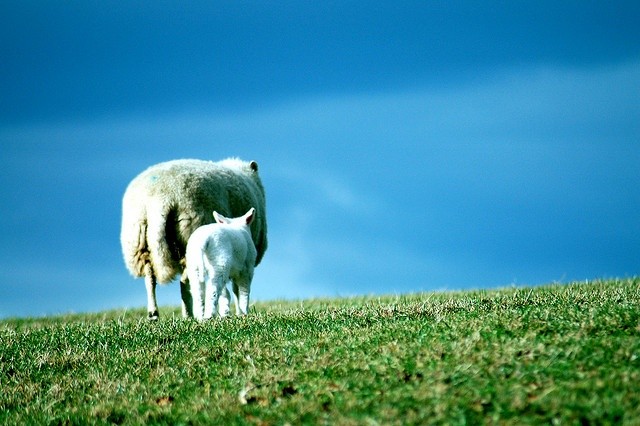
[185,207,258,321]
[120,157,268,325]
